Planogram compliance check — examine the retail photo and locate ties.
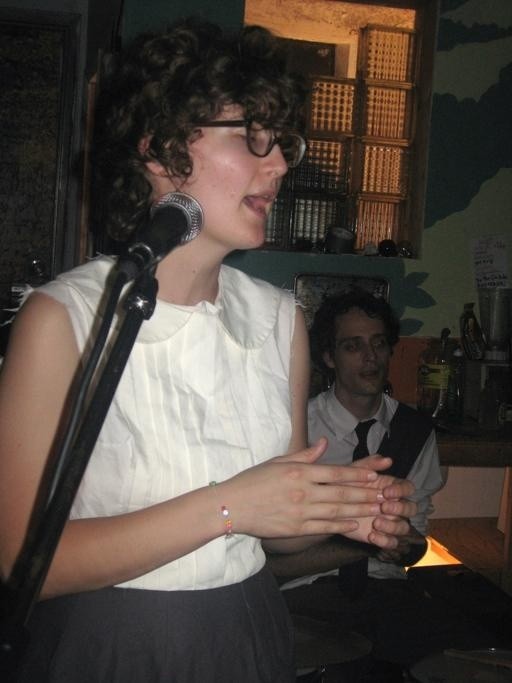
[338,418,378,603]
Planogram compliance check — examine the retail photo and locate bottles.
[417,338,457,417]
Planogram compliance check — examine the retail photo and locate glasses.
[191,115,309,170]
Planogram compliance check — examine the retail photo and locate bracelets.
[210,477,238,540]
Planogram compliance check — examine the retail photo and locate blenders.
[462,288,512,437]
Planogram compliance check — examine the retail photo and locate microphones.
[107,192,205,288]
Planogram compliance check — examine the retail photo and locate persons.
[1,18,417,683]
[304,286,445,539]
